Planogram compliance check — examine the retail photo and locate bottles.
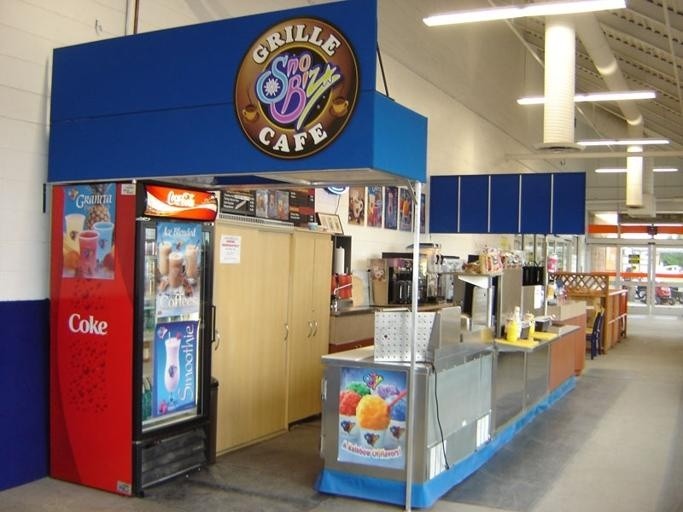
[505,306,535,343]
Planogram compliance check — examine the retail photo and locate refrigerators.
[42,180,218,495]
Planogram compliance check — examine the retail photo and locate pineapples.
[82,184,111,229]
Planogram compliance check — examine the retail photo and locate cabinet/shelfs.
[287,226,334,427]
[210,222,288,460]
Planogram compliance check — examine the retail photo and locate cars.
[663,265,681,274]
[655,287,675,304]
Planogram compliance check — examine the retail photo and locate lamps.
[594,156,680,174]
[537,13,585,155]
[421,1,628,28]
[517,19,659,106]
[625,143,644,208]
[573,86,671,146]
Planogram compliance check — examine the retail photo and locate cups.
[159,242,199,289]
[64,214,114,272]
[339,414,407,450]
[164,337,182,391]
[402,201,410,217]
[387,192,394,216]
[351,200,364,218]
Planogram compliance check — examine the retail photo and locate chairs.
[586,307,605,359]
